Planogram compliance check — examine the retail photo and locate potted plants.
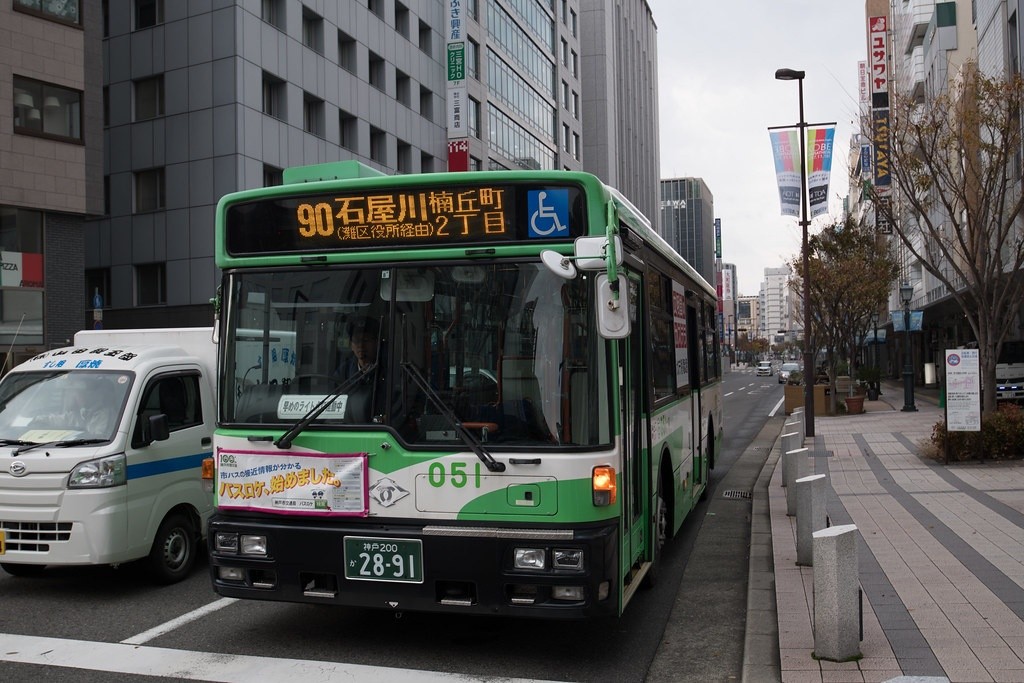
[783,360,881,414]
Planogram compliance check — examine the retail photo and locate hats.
[346,316,379,336]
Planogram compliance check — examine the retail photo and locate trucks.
[0,326,298,582]
[974,333,1024,406]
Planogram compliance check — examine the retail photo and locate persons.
[321,316,387,420]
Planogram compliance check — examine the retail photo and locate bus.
[200,158,731,621]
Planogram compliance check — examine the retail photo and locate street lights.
[775,68,815,436]
[898,281,917,413]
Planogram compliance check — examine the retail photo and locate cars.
[757,361,774,377]
[777,363,801,384]
